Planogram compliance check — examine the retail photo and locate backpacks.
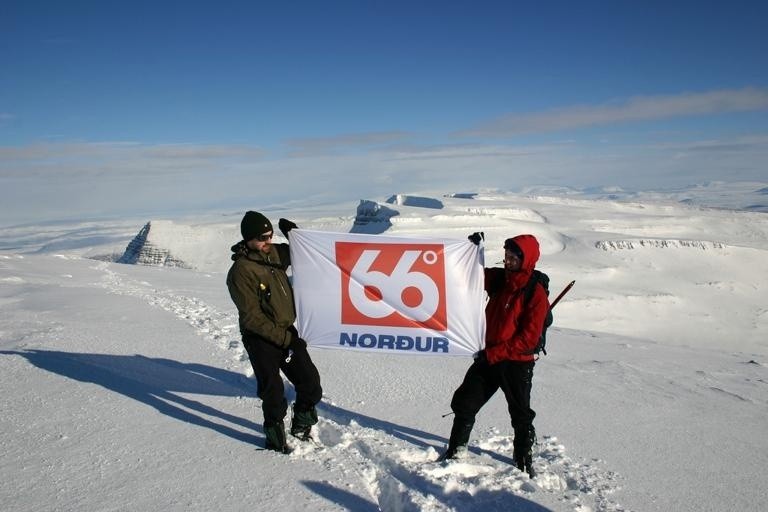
[526,269,554,356]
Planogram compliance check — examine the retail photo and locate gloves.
[278,218,298,240]
[474,350,492,369]
[468,231,487,245]
[289,334,308,355]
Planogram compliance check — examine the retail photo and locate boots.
[509,426,537,478]
[290,400,319,443]
[262,409,295,455]
[436,414,475,464]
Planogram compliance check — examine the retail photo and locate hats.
[240,211,273,241]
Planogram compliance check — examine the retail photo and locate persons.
[226,210,323,454]
[436,231,550,479]
